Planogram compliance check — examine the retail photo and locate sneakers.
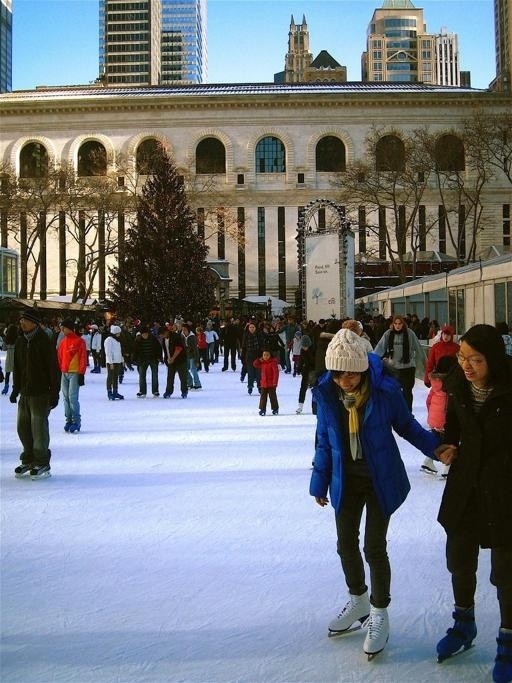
[137,391,187,397]
[109,393,124,400]
[16,464,33,473]
[31,464,50,475]
[64,423,80,431]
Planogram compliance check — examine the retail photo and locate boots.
[421,456,438,472]
[362,606,389,655]
[296,403,303,412]
[441,462,449,476]
[328,590,370,632]
[493,628,512,682]
[436,607,477,654]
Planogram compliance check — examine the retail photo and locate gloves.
[49,391,59,409]
[10,393,18,403]
[78,374,84,386]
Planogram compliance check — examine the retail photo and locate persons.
[1,306,467,479]
[306,318,458,657]
[432,324,512,682]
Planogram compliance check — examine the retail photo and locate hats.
[20,309,40,323]
[442,325,454,333]
[111,326,121,334]
[325,320,369,372]
[58,319,74,332]
[302,335,311,347]
[89,324,98,329]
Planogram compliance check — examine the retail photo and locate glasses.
[455,352,482,367]
